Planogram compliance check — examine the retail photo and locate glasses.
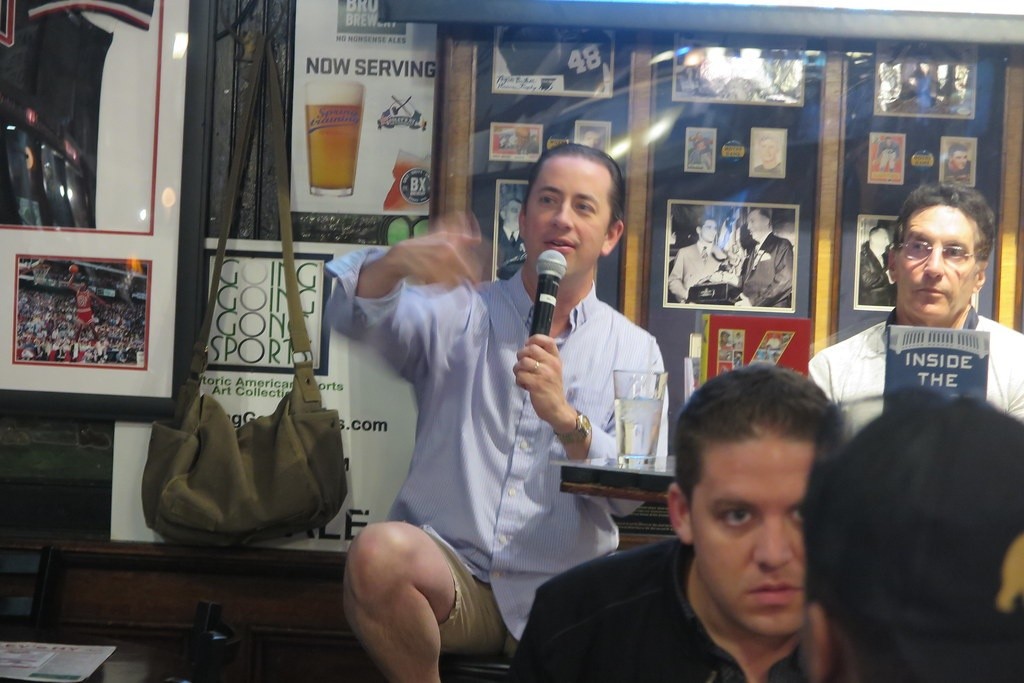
[897,241,981,263]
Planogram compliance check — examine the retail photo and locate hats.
[803,397,1024,683]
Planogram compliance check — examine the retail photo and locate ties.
[702,246,708,264]
[510,233,515,248]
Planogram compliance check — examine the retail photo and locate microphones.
[527,250,568,339]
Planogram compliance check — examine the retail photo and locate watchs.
[555,412,591,444]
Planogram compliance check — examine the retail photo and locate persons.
[943,144,971,184]
[757,333,783,363]
[669,207,792,308]
[808,184,1024,421]
[904,61,973,103]
[581,130,602,149]
[857,227,900,307]
[718,332,743,375]
[15,271,146,367]
[808,394,1024,683]
[319,144,670,683]
[505,360,849,683]
[689,132,712,169]
[755,135,781,174]
[499,192,521,259]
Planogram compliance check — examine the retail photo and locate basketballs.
[68,264,79,273]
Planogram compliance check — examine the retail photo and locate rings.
[531,361,539,373]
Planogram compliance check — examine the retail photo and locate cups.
[612,369,670,467]
[303,78,366,197]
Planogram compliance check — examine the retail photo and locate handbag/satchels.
[143,364,348,549]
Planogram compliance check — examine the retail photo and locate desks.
[558,457,677,507]
